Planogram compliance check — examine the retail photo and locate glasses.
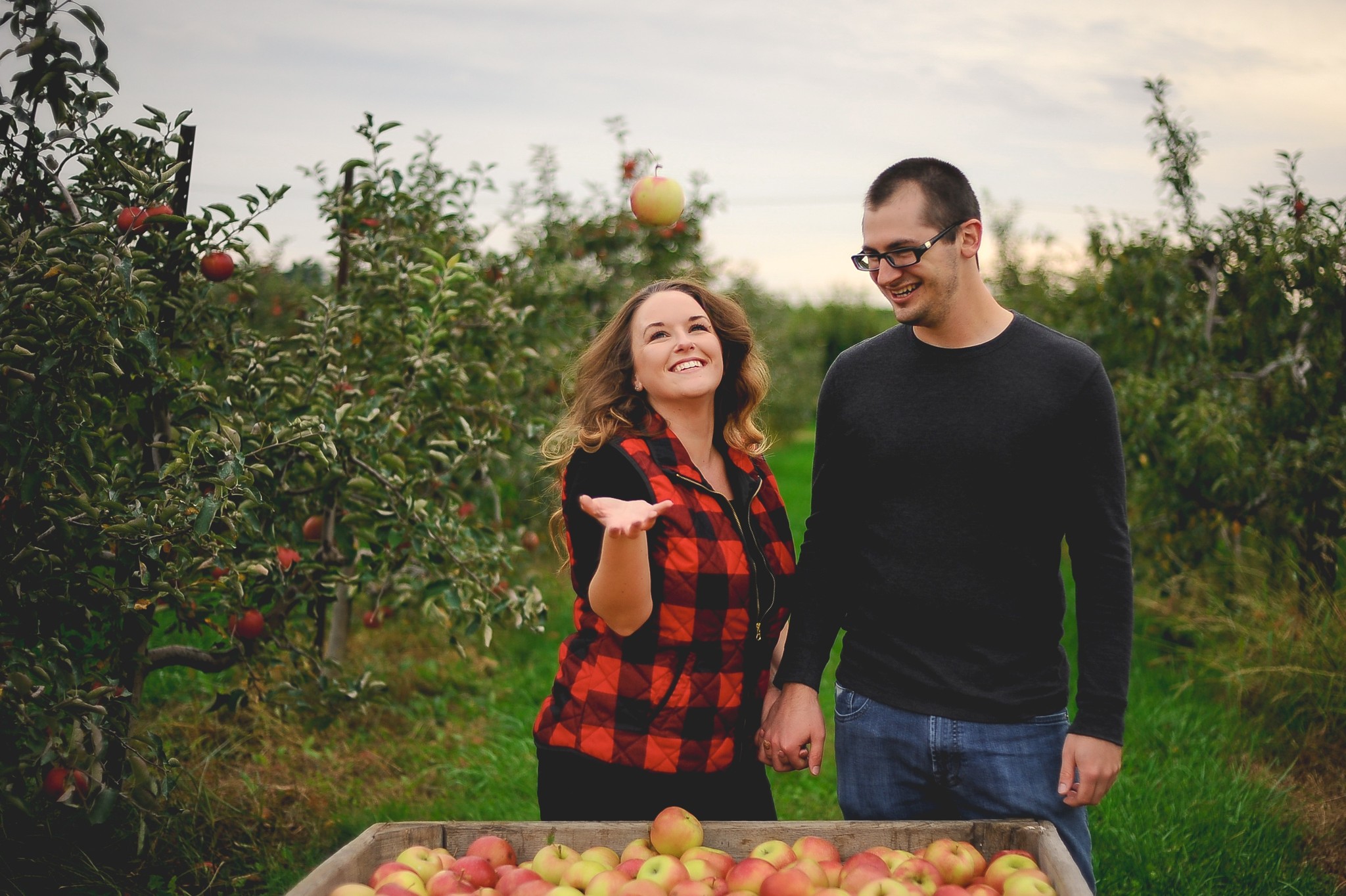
[851,220,962,272]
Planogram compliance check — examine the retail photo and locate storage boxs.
[286,818,1095,896]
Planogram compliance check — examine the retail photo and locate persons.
[531,281,811,820]
[759,157,1137,892]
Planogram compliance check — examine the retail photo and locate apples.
[46,200,687,808]
[630,165,684,225]
[195,862,219,876]
[624,160,639,178]
[329,806,1058,896]
